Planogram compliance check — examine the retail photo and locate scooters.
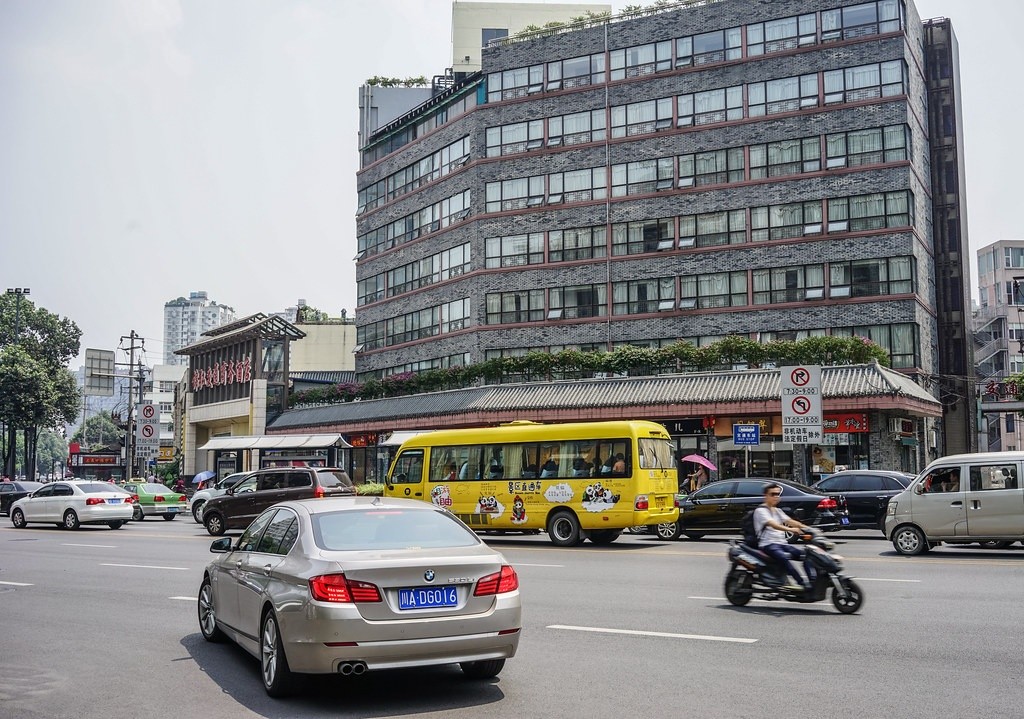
[724,529,863,613]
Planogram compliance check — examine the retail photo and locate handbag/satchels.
[690,477,696,491]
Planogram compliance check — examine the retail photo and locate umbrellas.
[191,470,216,487]
[681,453,717,474]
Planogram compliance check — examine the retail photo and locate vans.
[884,451,1024,556]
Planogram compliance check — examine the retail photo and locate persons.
[174,477,191,502]
[687,463,707,490]
[197,477,215,490]
[108,476,115,484]
[941,469,960,492]
[147,474,155,483]
[0,474,11,482]
[223,472,230,477]
[753,484,813,589]
[612,452,625,473]
[443,461,457,481]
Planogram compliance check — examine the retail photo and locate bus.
[381,421,683,547]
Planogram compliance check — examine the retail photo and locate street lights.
[52,459,55,482]
[62,462,64,480]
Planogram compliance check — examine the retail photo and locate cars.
[0,480,188,531]
[629,470,917,538]
[199,498,522,700]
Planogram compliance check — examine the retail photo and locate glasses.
[767,492,781,497]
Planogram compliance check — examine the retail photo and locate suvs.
[193,466,358,537]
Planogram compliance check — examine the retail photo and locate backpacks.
[740,505,770,548]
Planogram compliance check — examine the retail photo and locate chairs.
[1002,468,1017,489]
